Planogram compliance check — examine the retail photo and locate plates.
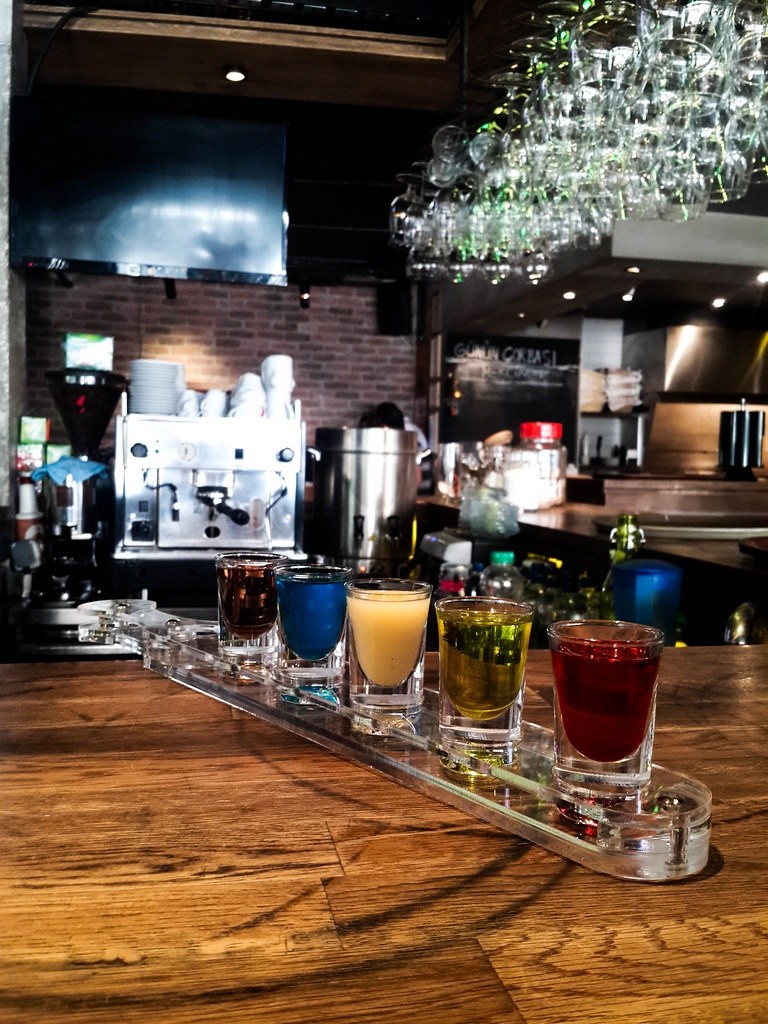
[127,359,185,414]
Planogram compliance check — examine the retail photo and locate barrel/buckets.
[306,428,438,559]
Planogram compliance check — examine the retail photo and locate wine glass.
[389,0,768,280]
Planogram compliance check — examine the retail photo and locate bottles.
[517,422,567,507]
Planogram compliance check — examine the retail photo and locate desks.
[304,473,768,594]
[0,646,768,1024]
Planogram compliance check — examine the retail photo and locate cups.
[273,564,353,679]
[341,577,433,710]
[434,596,534,745]
[215,551,287,654]
[181,355,296,419]
[547,621,663,803]
